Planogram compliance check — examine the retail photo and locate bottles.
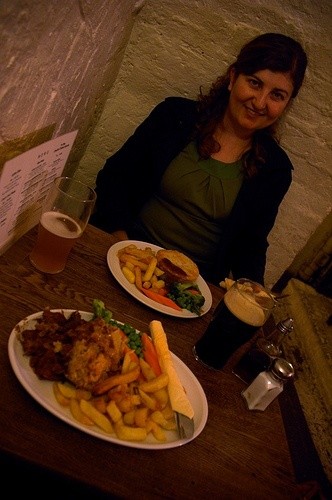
[241,357,292,411]
[232,317,293,383]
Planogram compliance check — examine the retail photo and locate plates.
[9,309,208,449]
[107,239,213,318]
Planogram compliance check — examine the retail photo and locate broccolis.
[89,298,142,357]
[168,281,207,316]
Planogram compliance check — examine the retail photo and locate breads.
[156,249,199,283]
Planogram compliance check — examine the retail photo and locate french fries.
[53,359,179,442]
[117,245,165,292]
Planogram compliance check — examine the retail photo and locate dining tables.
[0,222,296,500]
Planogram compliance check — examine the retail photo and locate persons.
[79,33,309,291]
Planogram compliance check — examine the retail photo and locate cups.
[30,176,97,274]
[193,278,277,367]
